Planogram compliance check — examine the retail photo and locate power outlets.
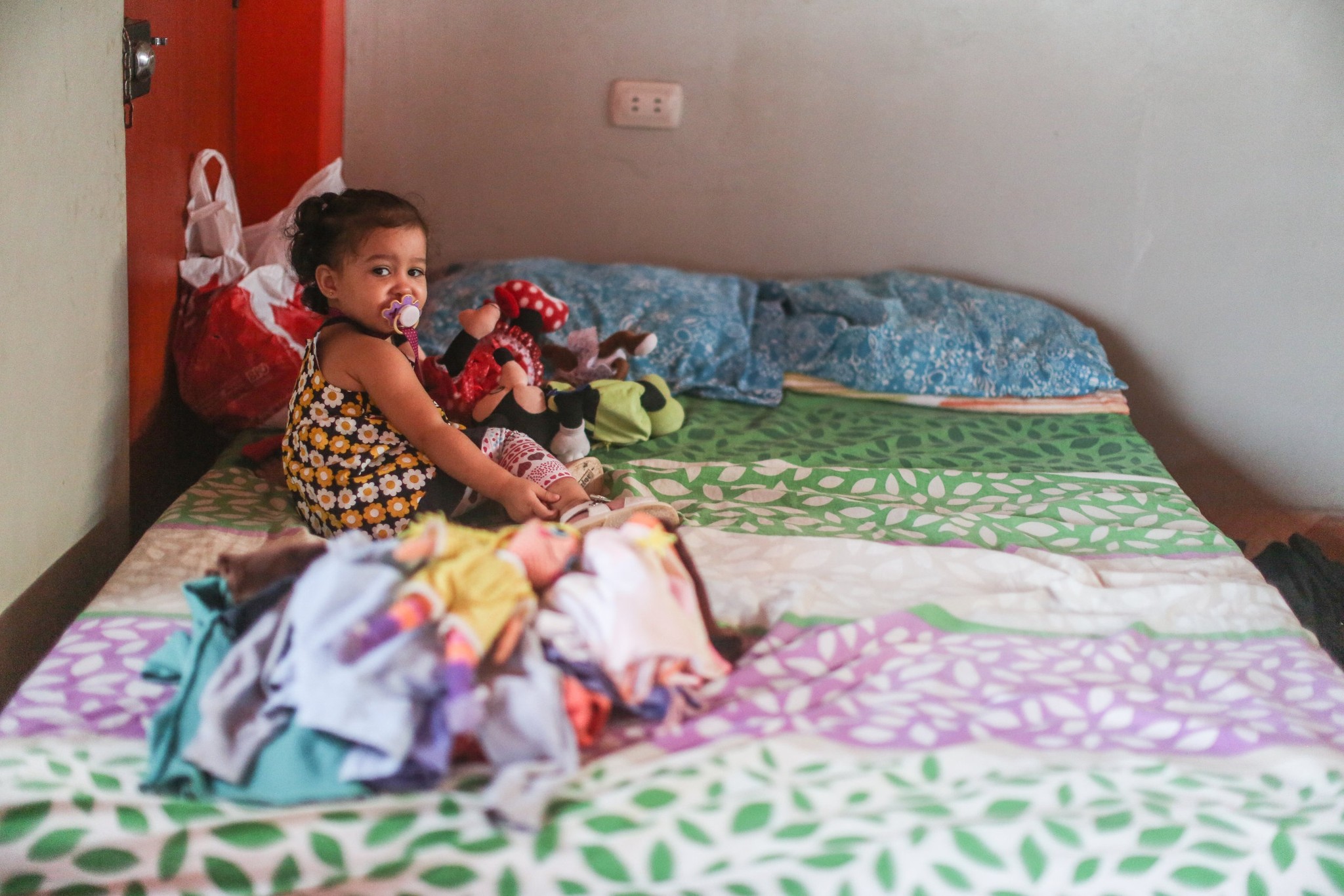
[609,80,682,129]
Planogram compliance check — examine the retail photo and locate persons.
[292,187,680,539]
[343,509,584,693]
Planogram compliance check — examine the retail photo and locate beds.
[0,256,1344,893]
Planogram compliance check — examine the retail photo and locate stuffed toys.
[418,279,684,464]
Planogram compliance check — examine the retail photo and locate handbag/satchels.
[172,143,355,427]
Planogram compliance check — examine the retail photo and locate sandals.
[558,492,681,538]
